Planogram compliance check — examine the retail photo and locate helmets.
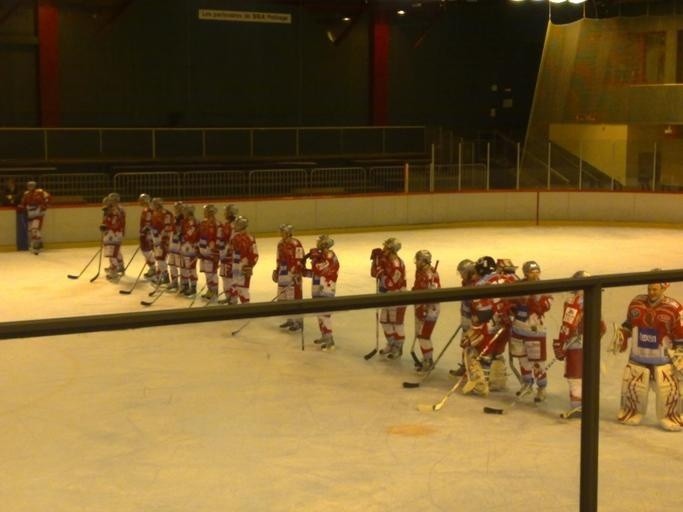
[414,249,432,265]
[102,192,120,205]
[280,223,294,236]
[26,181,36,189]
[456,255,540,278]
[572,270,591,278]
[139,193,163,207]
[650,269,671,288]
[316,234,334,250]
[384,237,401,253]
[172,202,249,231]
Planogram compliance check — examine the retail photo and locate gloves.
[553,338,568,361]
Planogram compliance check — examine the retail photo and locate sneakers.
[379,340,402,359]
[279,318,304,331]
[449,362,466,377]
[313,333,335,349]
[515,383,547,402]
[416,356,434,372]
[105,266,239,306]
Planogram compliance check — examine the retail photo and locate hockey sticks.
[410,261,439,369]
[508,330,522,384]
[482,337,575,415]
[227,286,287,336]
[218,296,232,305]
[415,327,506,411]
[299,253,309,352]
[65,235,197,307]
[402,325,461,388]
[364,256,379,362]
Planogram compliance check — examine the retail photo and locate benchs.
[1,150,468,211]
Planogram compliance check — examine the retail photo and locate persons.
[304,234,340,350]
[2,179,20,205]
[273,223,305,331]
[17,181,51,255]
[607,282,683,432]
[457,256,553,406]
[553,271,606,420]
[371,235,406,360]
[411,250,440,373]
[100,194,127,280]
[138,194,258,306]
